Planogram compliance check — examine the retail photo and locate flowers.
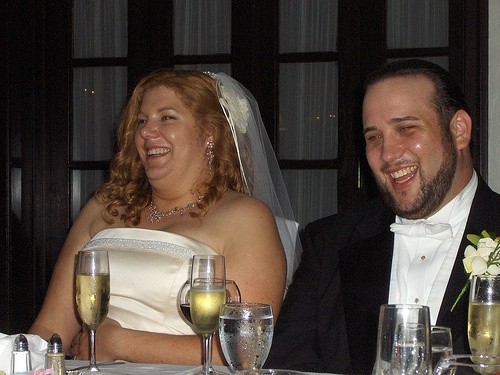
[218,85,249,134]
[451,230,500,312]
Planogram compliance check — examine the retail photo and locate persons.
[262,59,500,375]
[25,69,288,365]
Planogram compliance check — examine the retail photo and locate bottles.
[45,333,65,375]
[11,334,31,375]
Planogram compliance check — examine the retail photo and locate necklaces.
[144,194,205,224]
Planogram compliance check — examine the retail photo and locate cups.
[377,303,431,375]
[468,275,500,375]
[219,303,274,375]
[397,324,453,375]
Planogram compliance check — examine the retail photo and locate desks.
[64,360,342,375]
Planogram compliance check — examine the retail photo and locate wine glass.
[190,255,226,375]
[433,353,500,375]
[75,250,110,372]
[176,278,243,375]
